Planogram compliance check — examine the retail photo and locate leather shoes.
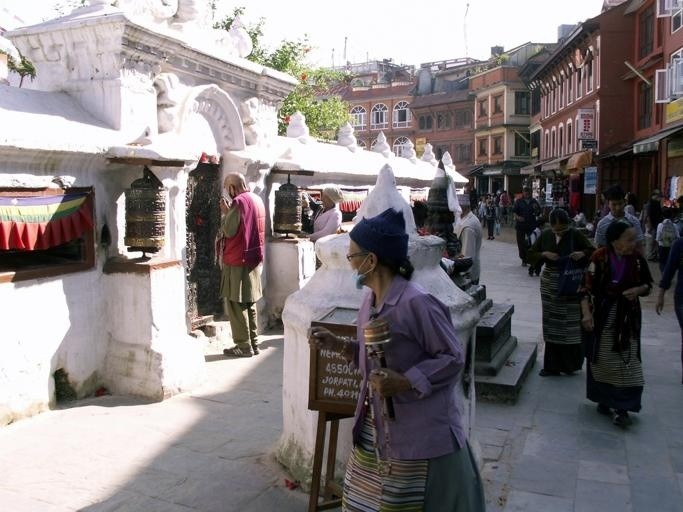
[613,414,633,426]
[597,405,615,416]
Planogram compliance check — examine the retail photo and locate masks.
[349,251,377,290]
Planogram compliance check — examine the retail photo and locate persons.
[346,206,485,511]
[512,187,548,276]
[593,191,645,253]
[453,195,483,285]
[531,209,593,377]
[624,190,683,274]
[655,237,683,330]
[301,194,322,234]
[218,172,266,357]
[576,221,655,427]
[412,199,448,260]
[469,190,510,240]
[297,185,343,270]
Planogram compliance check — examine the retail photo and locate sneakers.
[539,368,561,377]
[223,345,259,358]
[487,236,495,240]
[522,259,540,276]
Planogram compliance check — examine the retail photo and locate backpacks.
[661,222,677,247]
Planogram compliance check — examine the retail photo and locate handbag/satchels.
[496,224,501,236]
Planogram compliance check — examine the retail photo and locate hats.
[604,183,624,201]
[349,208,408,264]
[322,187,344,203]
[456,193,470,207]
